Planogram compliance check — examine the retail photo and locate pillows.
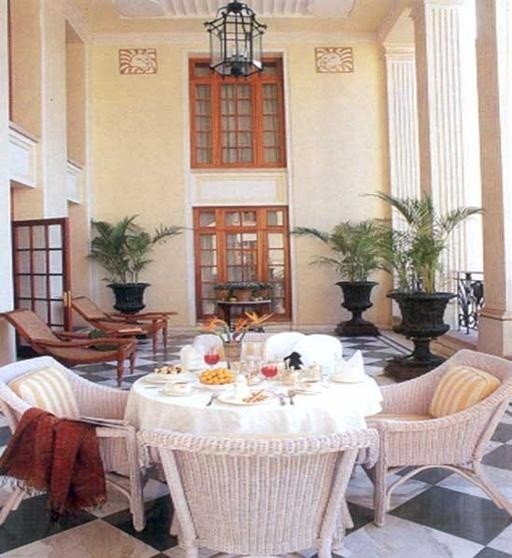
[427,366,501,419]
[8,366,79,421]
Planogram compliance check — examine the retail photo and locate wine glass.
[204,349,221,369]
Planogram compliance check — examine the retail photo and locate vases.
[223,340,241,361]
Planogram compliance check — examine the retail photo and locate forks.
[206,392,218,406]
[288,389,295,404]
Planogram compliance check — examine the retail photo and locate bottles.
[245,333,258,373]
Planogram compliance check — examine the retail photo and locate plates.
[162,387,199,396]
[197,379,238,391]
[218,388,270,405]
[143,366,194,384]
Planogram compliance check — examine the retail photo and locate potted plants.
[236,282,260,301]
[88,213,186,338]
[289,217,398,336]
[256,282,270,298]
[214,283,232,300]
[361,188,487,383]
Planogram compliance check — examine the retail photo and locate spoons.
[278,392,285,404]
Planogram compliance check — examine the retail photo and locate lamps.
[202,1,268,81]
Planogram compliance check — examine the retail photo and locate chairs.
[217,333,277,361]
[71,295,168,354]
[0,307,138,387]
[352,349,512,528]
[0,355,158,532]
[142,428,381,558]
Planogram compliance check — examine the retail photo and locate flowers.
[196,310,277,343]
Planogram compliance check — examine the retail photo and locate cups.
[261,363,278,392]
